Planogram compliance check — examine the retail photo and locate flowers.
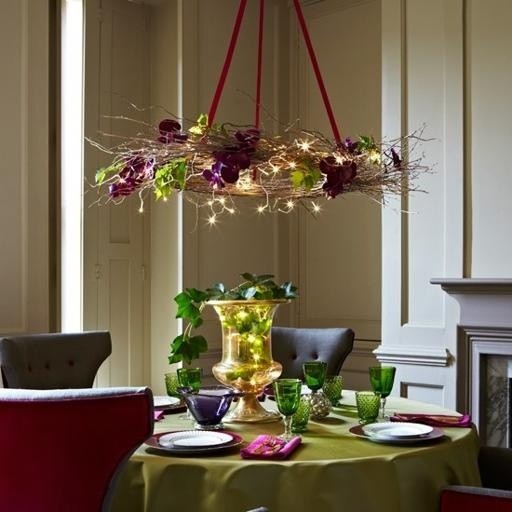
[76,88,439,234]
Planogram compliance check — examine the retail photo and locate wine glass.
[271,361,396,440]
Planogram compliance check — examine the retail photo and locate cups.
[163,366,203,406]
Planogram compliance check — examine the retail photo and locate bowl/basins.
[176,385,245,429]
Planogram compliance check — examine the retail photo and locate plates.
[348,420,445,446]
[143,430,244,454]
[151,395,187,412]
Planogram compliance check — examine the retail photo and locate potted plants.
[167,271,299,425]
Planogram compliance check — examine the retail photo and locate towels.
[390,413,472,428]
[240,434,303,461]
